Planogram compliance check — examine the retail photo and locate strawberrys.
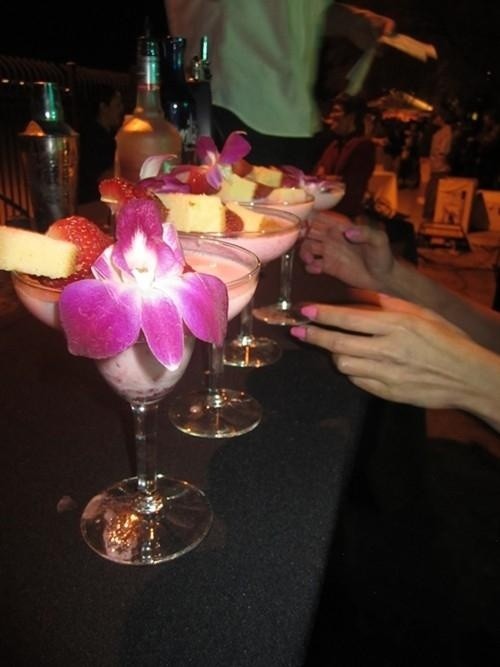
[40,216,117,288]
[232,158,253,176]
[98,175,169,223]
[222,202,245,238]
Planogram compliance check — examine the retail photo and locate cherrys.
[187,164,223,194]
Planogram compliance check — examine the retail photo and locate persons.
[156,0,397,168]
[312,97,499,210]
[289,211,500,433]
[77,81,125,203]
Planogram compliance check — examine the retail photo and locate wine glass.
[10,175,347,568]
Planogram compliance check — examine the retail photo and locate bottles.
[115,34,201,184]
[13,80,82,234]
